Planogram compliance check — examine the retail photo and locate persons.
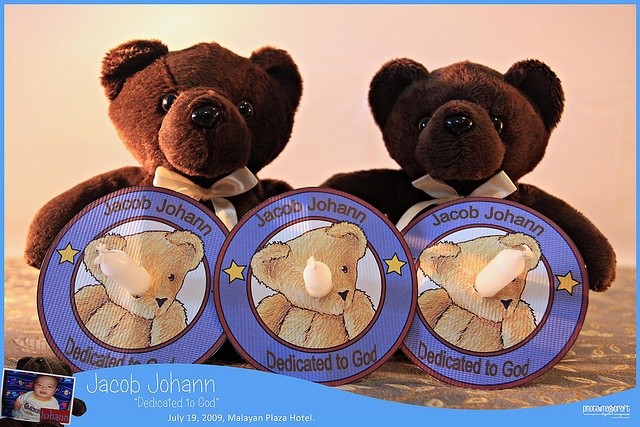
[13,374,61,422]
[0,356,87,427]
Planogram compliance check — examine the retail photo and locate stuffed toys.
[24,38,303,364]
[73,229,205,350]
[313,57,617,291]
[251,222,377,349]
[417,233,541,352]
